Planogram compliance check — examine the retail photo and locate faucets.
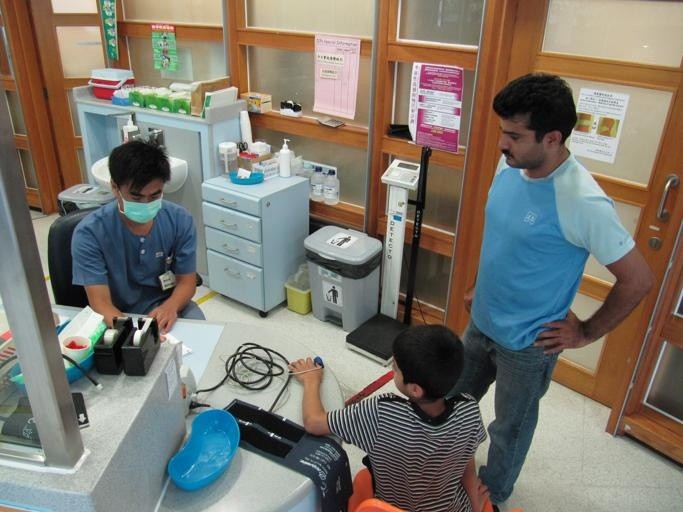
[131,128,165,151]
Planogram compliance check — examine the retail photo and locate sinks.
[91,153,190,194]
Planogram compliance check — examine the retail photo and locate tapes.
[133,318,153,346]
[103,317,128,345]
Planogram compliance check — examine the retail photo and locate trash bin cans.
[57,183,116,216]
[303,225,383,333]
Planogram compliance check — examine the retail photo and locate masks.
[123,198,162,223]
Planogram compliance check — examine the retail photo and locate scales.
[345,144,432,368]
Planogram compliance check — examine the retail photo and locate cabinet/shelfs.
[73,85,248,287]
[200,172,310,319]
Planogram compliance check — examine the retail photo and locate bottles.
[323,168,339,206]
[310,166,324,202]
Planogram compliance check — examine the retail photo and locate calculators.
[317,116,344,128]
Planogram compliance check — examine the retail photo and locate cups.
[219,140,237,180]
[63,336,92,367]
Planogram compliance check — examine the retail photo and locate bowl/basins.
[168,407,241,492]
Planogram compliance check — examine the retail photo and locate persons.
[69,139,207,334]
[336,233,351,247]
[328,286,338,303]
[284,321,492,512]
[446,69,658,506]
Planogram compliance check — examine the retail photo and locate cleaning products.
[310,164,339,203]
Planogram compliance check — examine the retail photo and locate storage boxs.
[237,152,273,172]
[252,158,280,180]
[190,78,231,117]
[88,67,135,100]
[129,89,191,115]
[240,90,271,114]
[284,281,312,315]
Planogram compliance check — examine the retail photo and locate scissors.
[237,142,248,153]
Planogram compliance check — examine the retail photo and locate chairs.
[348,468,494,512]
[47,207,203,308]
